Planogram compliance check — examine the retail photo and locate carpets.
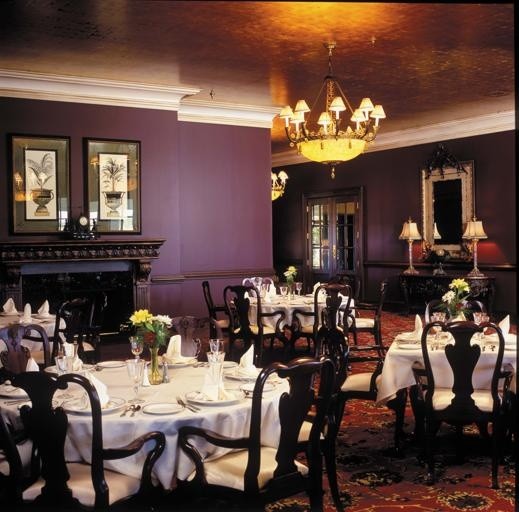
[194,310,519,512]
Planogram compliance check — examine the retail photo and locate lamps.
[277,40,386,180]
[399,216,422,273]
[271,171,288,201]
[434,223,442,239]
[462,216,488,277]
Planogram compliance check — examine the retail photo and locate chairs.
[202,280,236,352]
[242,277,274,298]
[349,281,388,357]
[292,284,352,350]
[171,317,223,361]
[0,368,166,512]
[409,322,516,489]
[297,326,349,512]
[329,274,360,318]
[22,299,101,370]
[321,306,408,460]
[223,284,289,367]
[0,324,51,372]
[425,299,487,328]
[177,360,335,512]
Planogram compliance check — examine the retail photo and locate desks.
[0,239,166,311]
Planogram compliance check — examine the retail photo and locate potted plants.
[101,158,126,218]
[27,153,55,216]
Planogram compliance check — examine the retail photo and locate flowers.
[463,240,475,253]
[433,278,475,322]
[431,249,449,263]
[283,266,299,285]
[423,240,434,253]
[129,308,173,349]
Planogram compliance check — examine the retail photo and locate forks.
[120,405,133,417]
[177,396,199,413]
[176,398,197,412]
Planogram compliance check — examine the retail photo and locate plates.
[226,374,257,381]
[223,361,238,368]
[0,388,29,399]
[98,361,125,367]
[45,365,56,374]
[399,343,422,350]
[159,356,198,365]
[143,402,184,414]
[242,382,278,392]
[62,397,125,413]
[186,389,246,403]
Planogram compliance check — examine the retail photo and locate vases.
[288,285,294,300]
[449,315,461,324]
[436,265,446,275]
[424,251,432,259]
[464,252,474,263]
[148,349,164,385]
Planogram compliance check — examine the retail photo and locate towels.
[499,315,510,334]
[240,344,255,376]
[201,366,228,401]
[38,299,50,317]
[26,359,39,373]
[166,335,182,361]
[63,343,83,370]
[415,314,423,337]
[3,299,17,313]
[24,304,31,317]
[313,282,320,293]
[82,373,108,409]
[264,292,271,303]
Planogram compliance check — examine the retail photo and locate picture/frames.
[7,133,72,236]
[82,137,142,233]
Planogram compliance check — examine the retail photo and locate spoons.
[130,405,141,417]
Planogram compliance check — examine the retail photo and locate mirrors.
[420,160,475,262]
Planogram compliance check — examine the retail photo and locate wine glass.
[280,287,287,301]
[130,336,143,360]
[431,316,444,340]
[207,351,225,379]
[296,283,302,297]
[56,356,73,376]
[473,312,488,340]
[433,312,446,316]
[126,360,146,404]
